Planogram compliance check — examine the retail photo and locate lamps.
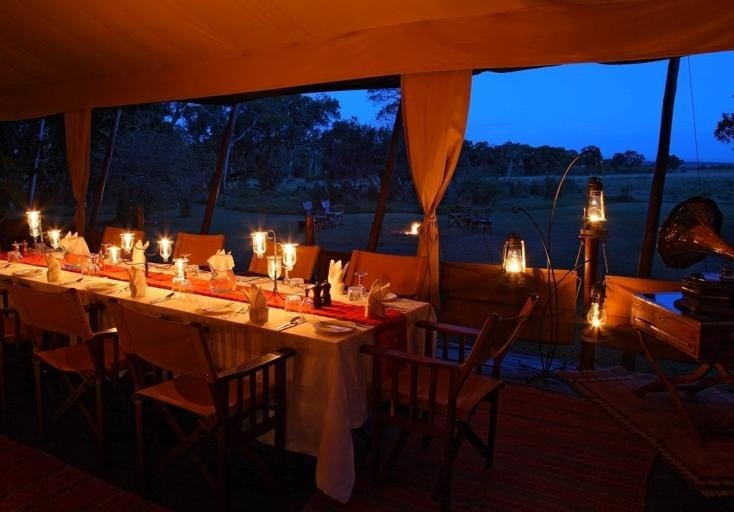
[497,143,616,389]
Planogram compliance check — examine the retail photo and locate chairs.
[301,198,327,232]
[321,199,345,228]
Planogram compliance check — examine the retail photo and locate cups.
[348,286,362,306]
[172,278,192,298]
[285,296,301,317]
[7,251,20,268]
[187,265,199,277]
[289,278,304,295]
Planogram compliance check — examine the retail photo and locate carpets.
[553,364,734,500]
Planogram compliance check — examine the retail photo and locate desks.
[624,283,734,449]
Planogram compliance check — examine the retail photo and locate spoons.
[151,293,174,305]
[61,278,84,286]
[274,315,305,331]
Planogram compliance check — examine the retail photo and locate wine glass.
[298,283,316,319]
[354,271,368,298]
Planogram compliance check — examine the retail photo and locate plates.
[315,320,356,333]
[12,269,42,276]
[200,302,236,313]
[383,293,397,300]
[85,282,117,289]
